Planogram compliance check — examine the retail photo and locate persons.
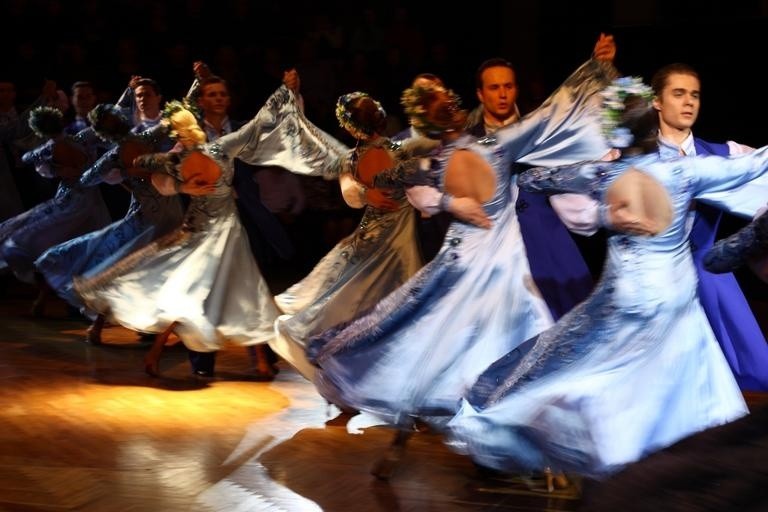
[512,79,767,511]
[8,63,295,383]
[548,63,767,496]
[270,33,617,497]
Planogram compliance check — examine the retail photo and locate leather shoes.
[28,299,279,381]
[311,401,584,494]
